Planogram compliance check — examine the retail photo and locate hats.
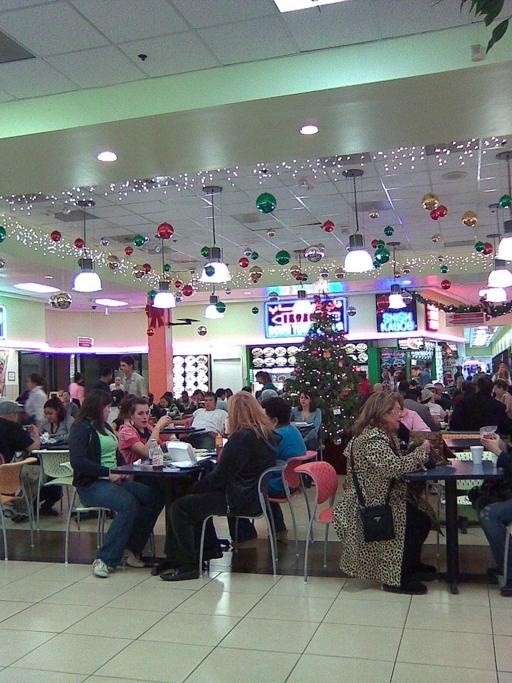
[0,400,24,416]
[421,383,435,402]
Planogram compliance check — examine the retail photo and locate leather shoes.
[160,568,199,581]
[152,559,177,576]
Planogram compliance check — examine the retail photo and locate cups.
[471,446,484,465]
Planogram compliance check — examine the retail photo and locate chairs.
[436,448,498,558]
[195,430,348,585]
[1,424,162,568]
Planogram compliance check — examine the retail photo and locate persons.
[1,357,321,581]
[331,363,511,594]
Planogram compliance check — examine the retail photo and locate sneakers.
[231,538,257,550]
[383,580,426,594]
[487,567,512,596]
[406,563,435,572]
[123,549,145,568]
[91,558,108,578]
[273,528,288,545]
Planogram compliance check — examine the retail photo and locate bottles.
[215,434,223,455]
[148,439,165,469]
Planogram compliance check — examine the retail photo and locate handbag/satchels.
[468,478,512,509]
[360,504,395,542]
[407,431,456,466]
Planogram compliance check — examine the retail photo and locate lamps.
[341,169,375,273]
[484,150,512,302]
[205,282,226,319]
[71,200,102,291]
[386,242,407,309]
[294,249,314,314]
[152,234,176,309]
[198,186,230,283]
